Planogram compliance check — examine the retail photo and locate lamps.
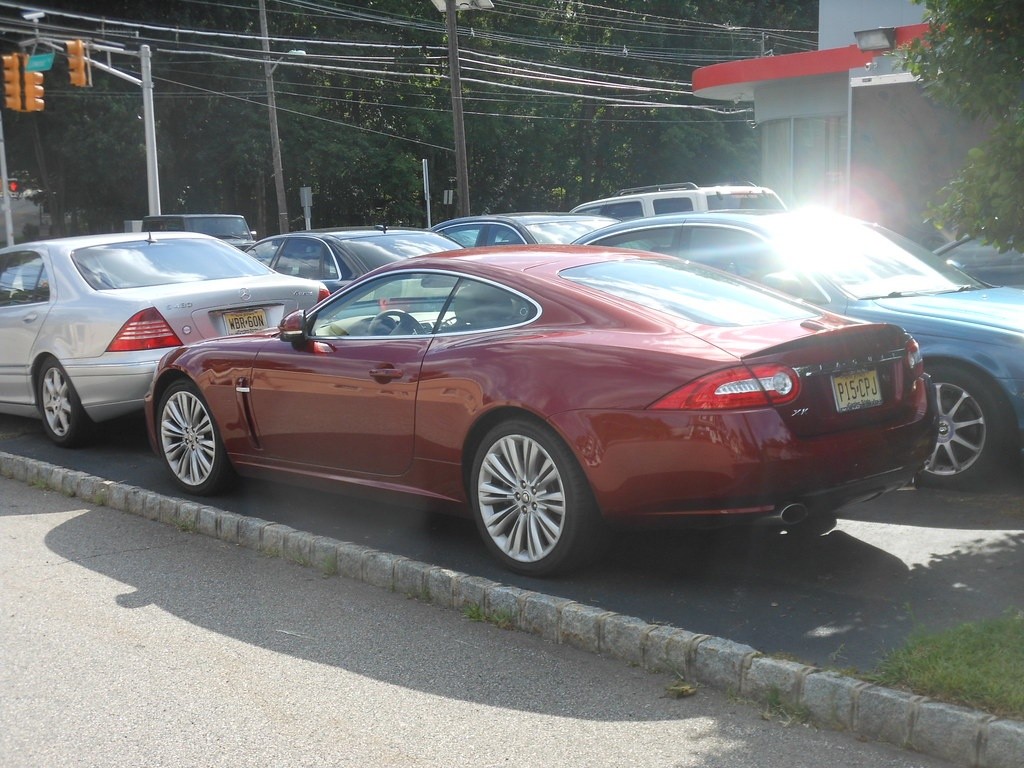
[854,27,896,54]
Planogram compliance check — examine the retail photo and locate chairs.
[448,285,513,329]
[299,256,329,279]
[12,264,49,303]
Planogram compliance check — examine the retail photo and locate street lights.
[267,50,307,235]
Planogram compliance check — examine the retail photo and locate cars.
[570,209,1024,492]
[142,180,789,296]
[144,246,940,579]
[1,232,330,449]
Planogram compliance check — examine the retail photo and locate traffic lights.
[23,54,44,111]
[63,39,86,86]
[8,178,21,198]
[2,53,22,111]
